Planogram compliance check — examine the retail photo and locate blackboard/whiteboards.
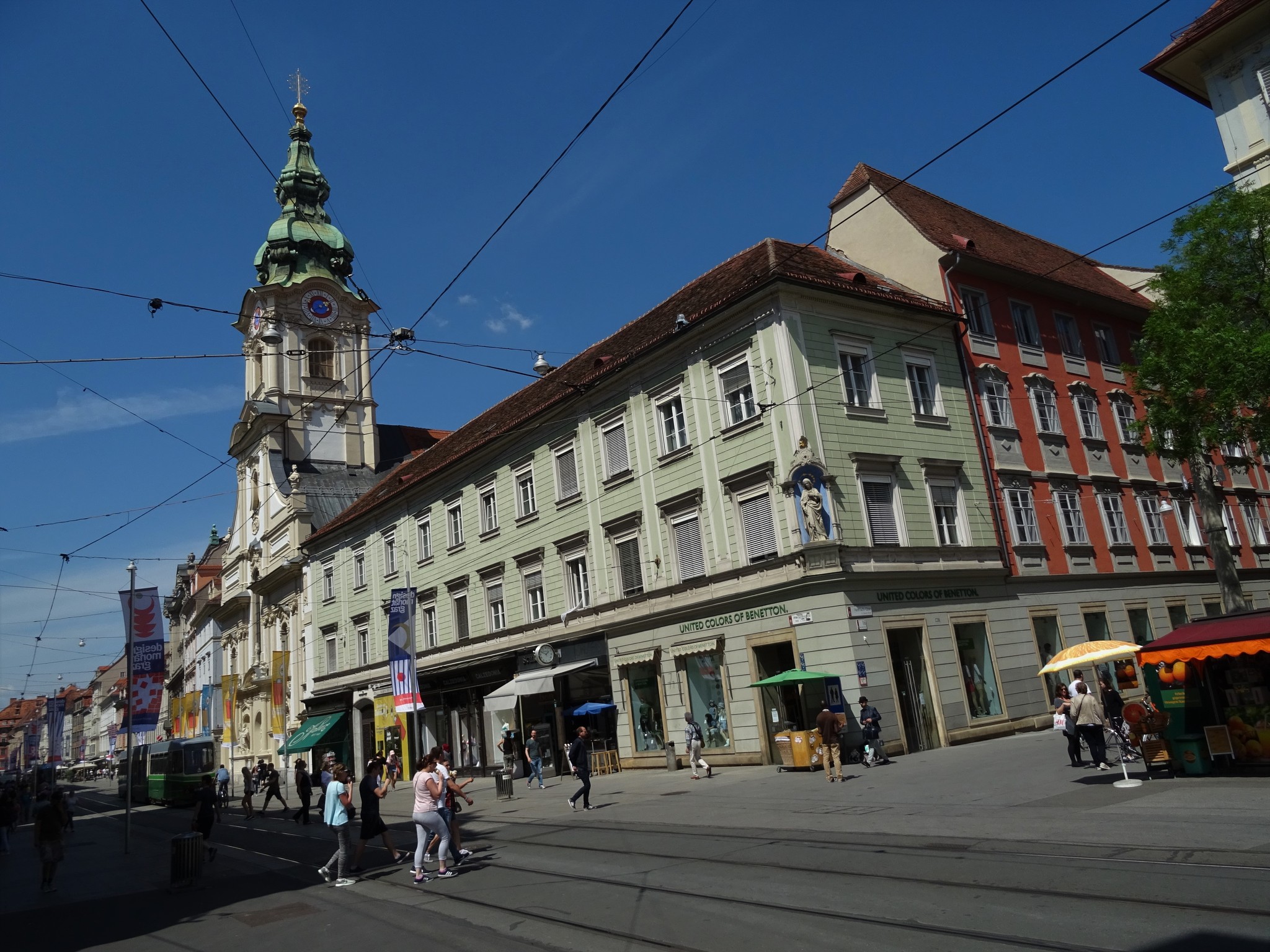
[1204,725,1234,755]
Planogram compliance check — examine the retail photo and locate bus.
[118,735,217,808]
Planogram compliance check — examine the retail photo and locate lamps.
[530,351,549,374]
[282,555,294,571]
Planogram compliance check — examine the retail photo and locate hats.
[857,696,868,702]
[717,702,725,709]
[390,750,395,755]
[709,700,715,703]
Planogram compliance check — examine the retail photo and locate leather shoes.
[691,776,699,779]
[706,767,711,778]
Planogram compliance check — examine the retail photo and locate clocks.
[249,297,266,336]
[531,642,563,664]
[297,288,339,325]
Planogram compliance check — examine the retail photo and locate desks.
[583,737,613,776]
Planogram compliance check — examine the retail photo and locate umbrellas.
[1036,637,1147,789]
[741,667,848,730]
[560,698,618,751]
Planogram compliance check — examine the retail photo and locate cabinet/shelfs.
[533,728,553,768]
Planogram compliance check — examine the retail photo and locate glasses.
[1060,688,1067,691]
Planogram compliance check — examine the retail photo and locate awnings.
[1133,612,1270,670]
[275,707,348,756]
[482,666,553,713]
[512,656,600,697]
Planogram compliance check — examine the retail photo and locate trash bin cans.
[166,831,205,895]
[1175,733,1208,776]
[664,740,678,771]
[495,768,513,799]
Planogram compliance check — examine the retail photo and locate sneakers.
[539,785,546,789]
[861,761,870,768]
[705,737,730,747]
[838,778,846,782]
[200,784,474,888]
[977,711,992,717]
[568,798,576,810]
[527,781,531,788]
[828,778,834,783]
[1071,737,1143,770]
[583,804,597,810]
[880,759,890,765]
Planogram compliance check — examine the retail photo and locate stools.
[603,749,621,775]
[591,751,609,777]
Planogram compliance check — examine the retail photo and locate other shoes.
[41,883,58,893]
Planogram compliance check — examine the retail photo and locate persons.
[800,478,830,543]
[1069,681,1112,771]
[1096,661,1116,690]
[652,700,667,750]
[704,713,719,742]
[500,714,510,732]
[1068,669,1093,751]
[1042,642,1063,702]
[33,790,71,895]
[526,729,547,790]
[496,730,517,780]
[1052,682,1091,768]
[1097,677,1135,761]
[0,754,120,857]
[682,711,712,780]
[637,696,661,750]
[959,652,987,718]
[568,724,598,812]
[717,702,731,747]
[966,654,993,716]
[190,732,480,888]
[858,695,890,768]
[707,699,719,727]
[816,699,848,783]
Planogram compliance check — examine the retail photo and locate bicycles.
[213,780,229,810]
[1087,690,1162,766]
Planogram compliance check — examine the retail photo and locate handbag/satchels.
[396,763,400,776]
[862,722,879,740]
[1053,711,1068,732]
[699,738,705,748]
[1121,720,1130,739]
